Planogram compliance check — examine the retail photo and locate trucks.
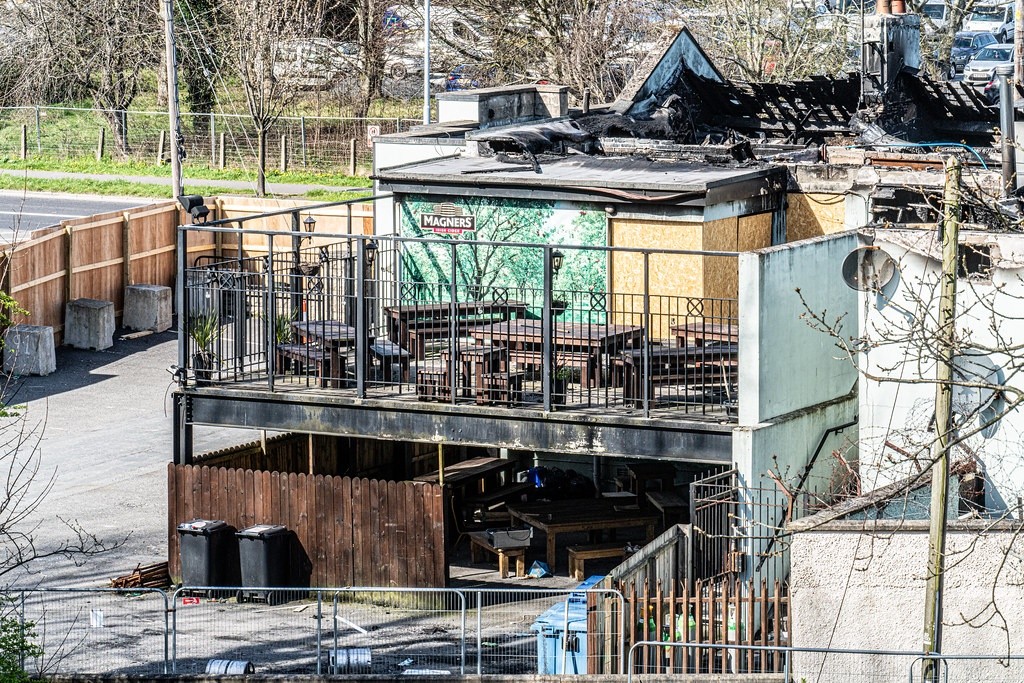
[383,4,494,82]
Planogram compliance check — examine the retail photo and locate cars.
[963,43,1016,86]
[957,4,1016,44]
[533,2,860,82]
[918,2,953,33]
[248,37,382,101]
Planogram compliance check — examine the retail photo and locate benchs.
[408,326,468,361]
[481,372,524,409]
[505,349,594,389]
[276,343,347,388]
[610,356,623,388]
[370,338,412,385]
[642,374,738,408]
[409,318,502,328]
[646,491,689,531]
[468,531,525,575]
[476,483,534,505]
[417,368,447,401]
[567,542,624,581]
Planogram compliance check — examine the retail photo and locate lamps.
[552,248,565,277]
[366,242,378,267]
[298,212,316,246]
[177,193,211,226]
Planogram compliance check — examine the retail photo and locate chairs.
[451,496,487,549]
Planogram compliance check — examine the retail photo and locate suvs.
[932,30,1001,80]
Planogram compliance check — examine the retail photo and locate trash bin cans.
[234,524,291,606]
[176,516,237,601]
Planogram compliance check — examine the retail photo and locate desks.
[292,320,375,388]
[439,345,506,404]
[506,500,659,573]
[468,318,644,389]
[413,457,518,498]
[384,301,529,351]
[671,322,739,348]
[618,345,738,409]
[626,464,667,496]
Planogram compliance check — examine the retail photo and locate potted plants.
[552,348,578,405]
[178,307,231,387]
[553,293,572,315]
[260,307,300,374]
